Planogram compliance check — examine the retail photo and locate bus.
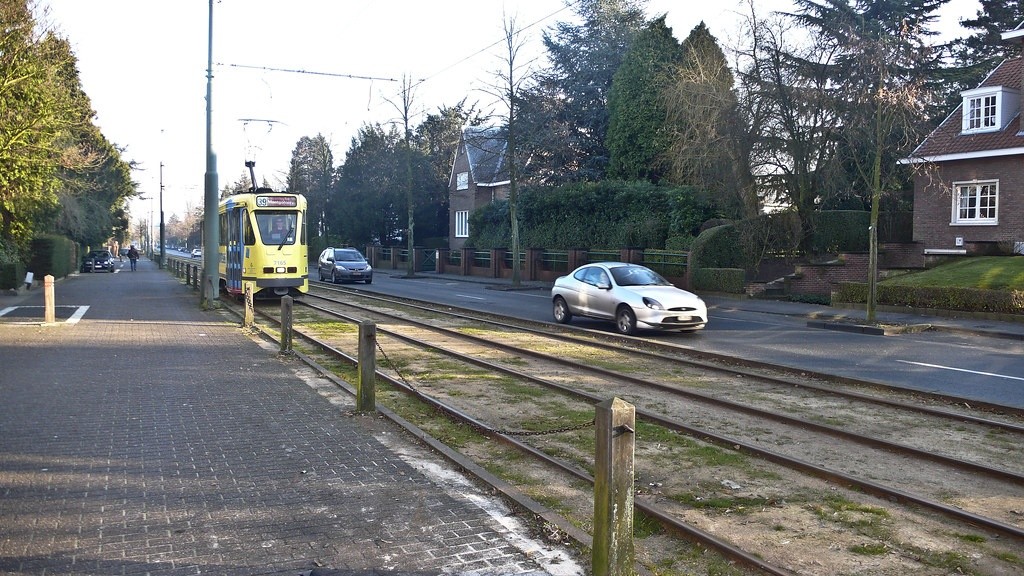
[200,191,310,301]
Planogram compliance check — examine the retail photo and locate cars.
[190,248,201,258]
[549,260,709,335]
[81,250,116,273]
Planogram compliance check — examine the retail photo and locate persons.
[127,245,139,271]
[271,218,287,238]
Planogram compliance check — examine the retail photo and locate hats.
[276,219,283,222]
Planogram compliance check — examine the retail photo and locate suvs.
[317,245,373,285]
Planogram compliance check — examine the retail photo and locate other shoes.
[134,267,137,271]
